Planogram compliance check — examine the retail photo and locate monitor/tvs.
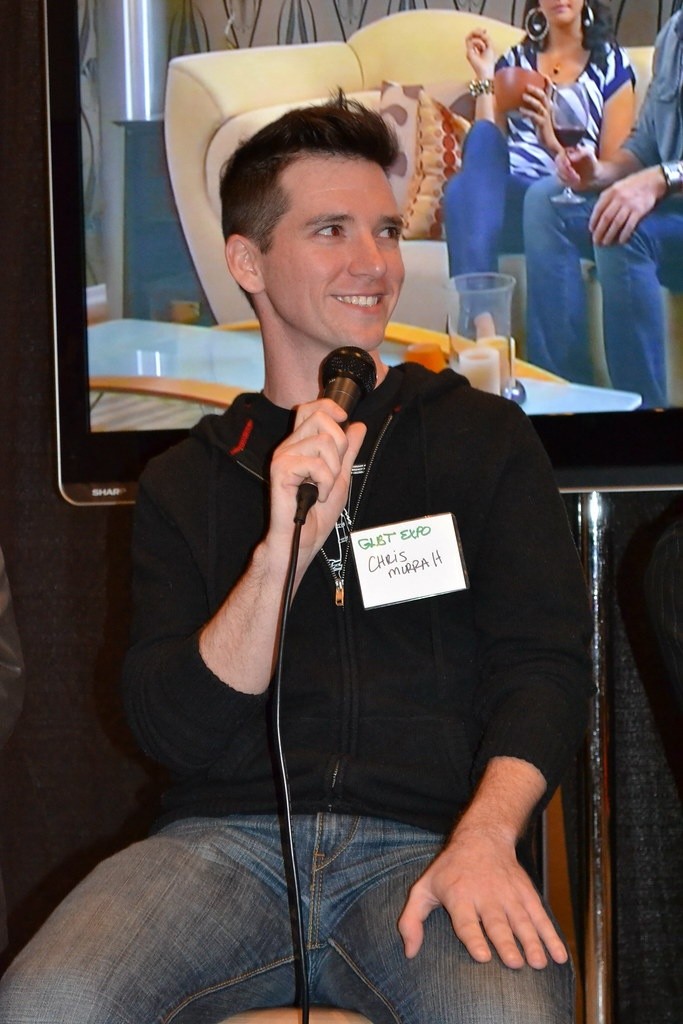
[41,0,683,505]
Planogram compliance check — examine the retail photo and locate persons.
[0,84,599,1024]
[442,1,683,406]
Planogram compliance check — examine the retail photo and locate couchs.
[163,8,683,407]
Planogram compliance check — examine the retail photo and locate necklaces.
[554,63,560,74]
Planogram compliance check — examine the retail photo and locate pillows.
[397,84,476,239]
[378,78,479,212]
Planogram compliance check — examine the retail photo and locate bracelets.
[466,76,494,97]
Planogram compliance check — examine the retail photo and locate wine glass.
[549,85,589,205]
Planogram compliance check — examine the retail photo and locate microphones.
[293,344,377,524]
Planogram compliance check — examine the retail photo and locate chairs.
[217,788,576,1024]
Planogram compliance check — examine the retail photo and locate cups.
[444,272,515,393]
